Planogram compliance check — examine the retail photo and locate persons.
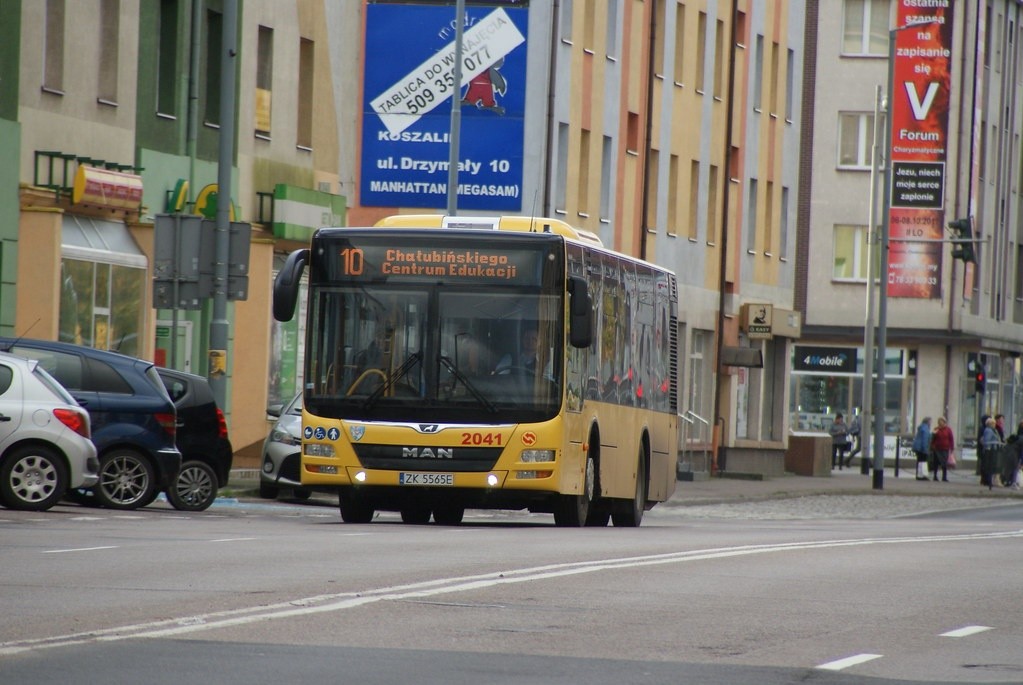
[976,415,1022,489]
[933,418,955,482]
[845,406,874,470]
[829,411,849,471]
[911,417,938,480]
[489,329,554,384]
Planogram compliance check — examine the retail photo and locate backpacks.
[850,416,860,441]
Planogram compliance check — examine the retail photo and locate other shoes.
[839,464,842,470]
[831,464,834,469]
[933,476,948,482]
[979,480,1021,490]
[845,460,851,468]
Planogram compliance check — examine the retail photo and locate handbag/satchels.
[918,455,929,478]
[845,435,852,453]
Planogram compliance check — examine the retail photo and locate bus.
[268,212,682,530]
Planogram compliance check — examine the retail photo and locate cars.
[0,348,101,513]
[259,388,341,502]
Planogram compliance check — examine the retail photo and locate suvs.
[0,335,183,511]
[154,366,233,512]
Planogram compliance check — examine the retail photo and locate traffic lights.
[948,216,979,266]
[975,362,986,394]
